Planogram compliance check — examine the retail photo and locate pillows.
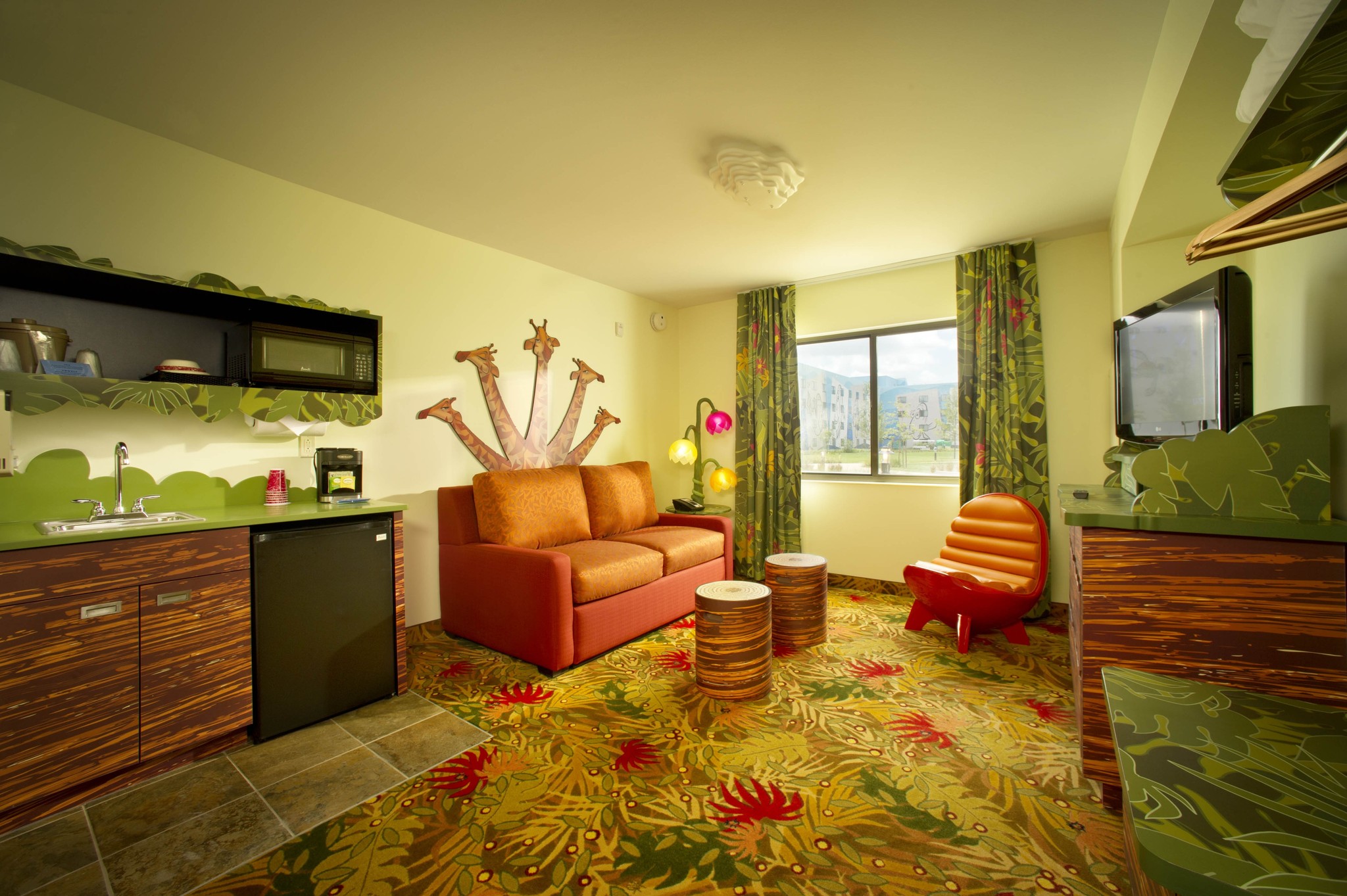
[472,465,591,551]
[579,461,660,541]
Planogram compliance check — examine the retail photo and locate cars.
[855,443,871,449]
[819,445,841,450]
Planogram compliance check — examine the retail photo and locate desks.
[1100,664,1347,896]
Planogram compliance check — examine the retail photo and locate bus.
[913,439,937,450]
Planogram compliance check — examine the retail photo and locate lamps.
[711,146,801,207]
[668,397,738,511]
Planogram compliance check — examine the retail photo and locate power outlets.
[298,436,316,458]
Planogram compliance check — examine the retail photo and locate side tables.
[666,504,731,517]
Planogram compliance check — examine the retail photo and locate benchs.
[434,460,736,671]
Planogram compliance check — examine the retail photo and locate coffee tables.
[694,579,773,702]
[764,553,829,650]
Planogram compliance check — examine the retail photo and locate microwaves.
[226,320,375,392]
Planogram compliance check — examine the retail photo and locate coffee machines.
[313,447,363,503]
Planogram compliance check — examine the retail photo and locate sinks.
[33,511,206,536]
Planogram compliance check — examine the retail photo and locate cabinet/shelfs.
[0,528,255,837]
[1056,484,1347,811]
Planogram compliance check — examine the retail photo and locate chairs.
[904,493,1051,649]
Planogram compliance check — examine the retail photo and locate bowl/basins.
[0,318,73,374]
[154,359,212,375]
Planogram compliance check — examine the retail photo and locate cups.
[266,506,288,517]
[264,469,288,506]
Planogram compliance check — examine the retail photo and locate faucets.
[113,441,130,514]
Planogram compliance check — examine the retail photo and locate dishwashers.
[246,519,394,746]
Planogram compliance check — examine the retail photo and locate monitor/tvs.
[1114,265,1253,449]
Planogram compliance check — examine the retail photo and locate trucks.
[937,440,952,448]
